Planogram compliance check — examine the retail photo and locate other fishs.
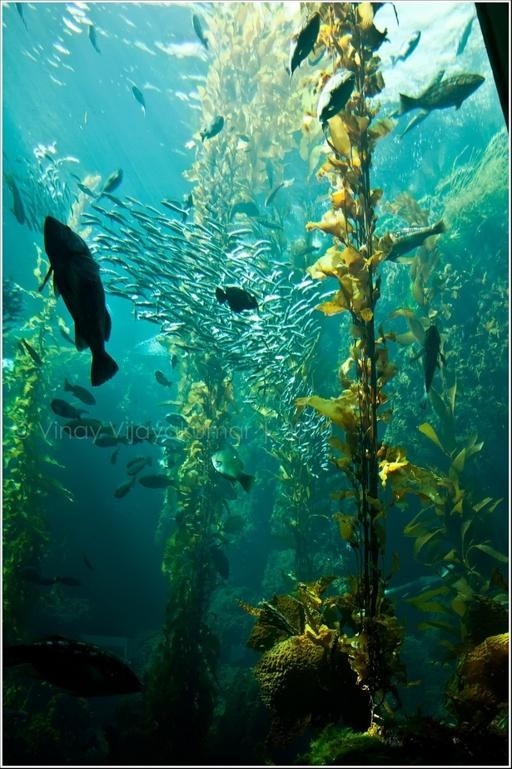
[379,219,446,264]
[409,322,447,392]
[2,142,331,588]
